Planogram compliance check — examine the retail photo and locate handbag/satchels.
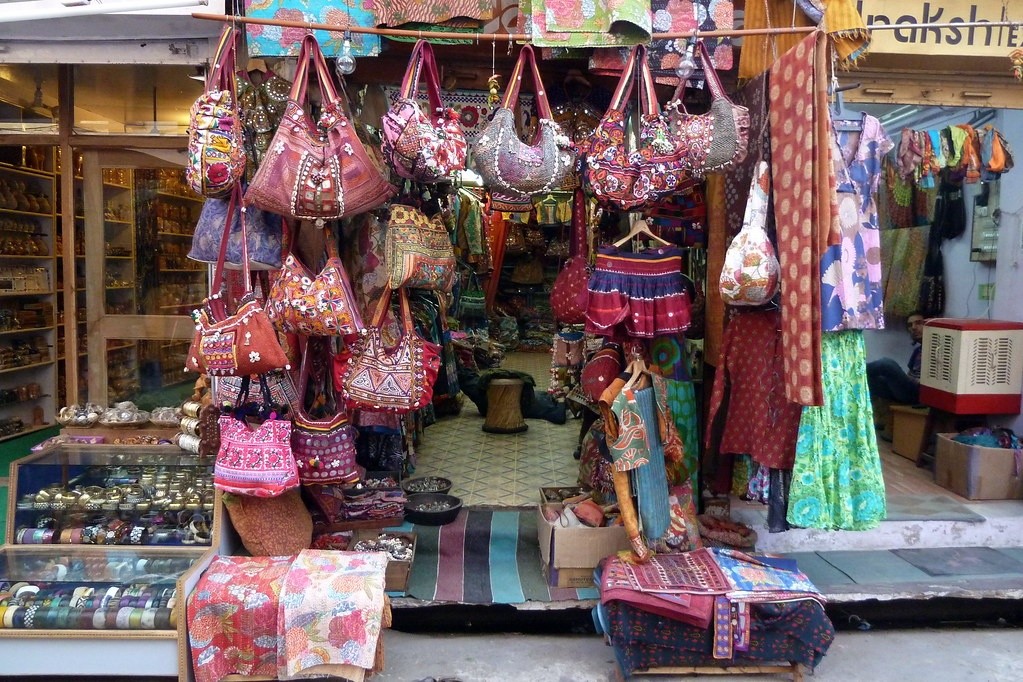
[474,44,576,199]
[583,40,751,210]
[188,25,467,557]
[551,189,589,325]
[720,161,782,307]
[505,221,570,285]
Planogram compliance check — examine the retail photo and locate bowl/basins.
[403,477,453,497]
[402,493,464,526]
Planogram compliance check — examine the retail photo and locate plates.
[55,414,180,428]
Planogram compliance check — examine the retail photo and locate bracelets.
[0,402,217,628]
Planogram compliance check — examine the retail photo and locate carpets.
[882,493,986,522]
[888,546,1022,577]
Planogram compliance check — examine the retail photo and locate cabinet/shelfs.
[0,143,234,679]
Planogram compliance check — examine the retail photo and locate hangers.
[624,346,651,390]
[830,90,862,131]
[614,219,670,247]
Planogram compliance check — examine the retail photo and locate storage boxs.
[537,486,634,588]
[890,404,929,460]
[347,531,420,590]
[935,431,1022,500]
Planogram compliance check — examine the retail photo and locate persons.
[867,309,929,403]
